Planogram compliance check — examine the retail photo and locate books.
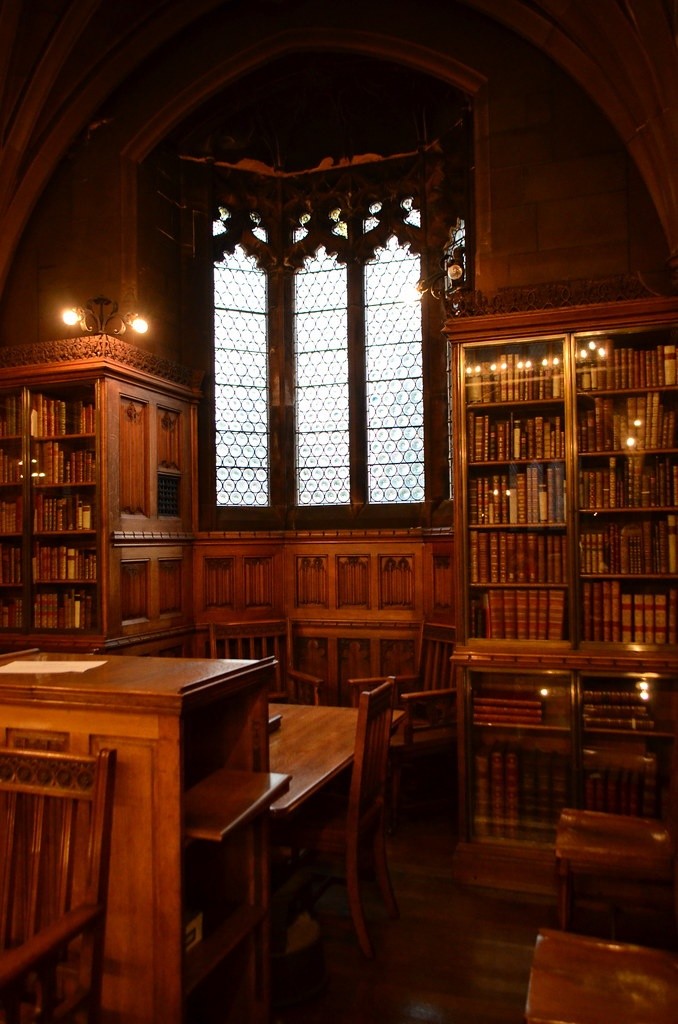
[1,393,99,628]
[180,767,292,841]
[466,345,677,841]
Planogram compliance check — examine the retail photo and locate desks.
[269,700,406,958]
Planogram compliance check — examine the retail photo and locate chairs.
[343,617,456,836]
[553,747,678,929]
[288,677,402,956]
[525,929,678,1024]
[0,744,114,1024]
[207,621,323,705]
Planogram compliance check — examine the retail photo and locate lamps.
[60,295,149,336]
[412,244,465,301]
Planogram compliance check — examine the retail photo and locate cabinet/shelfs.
[0,334,201,659]
[0,652,294,1024]
[450,292,678,912]
